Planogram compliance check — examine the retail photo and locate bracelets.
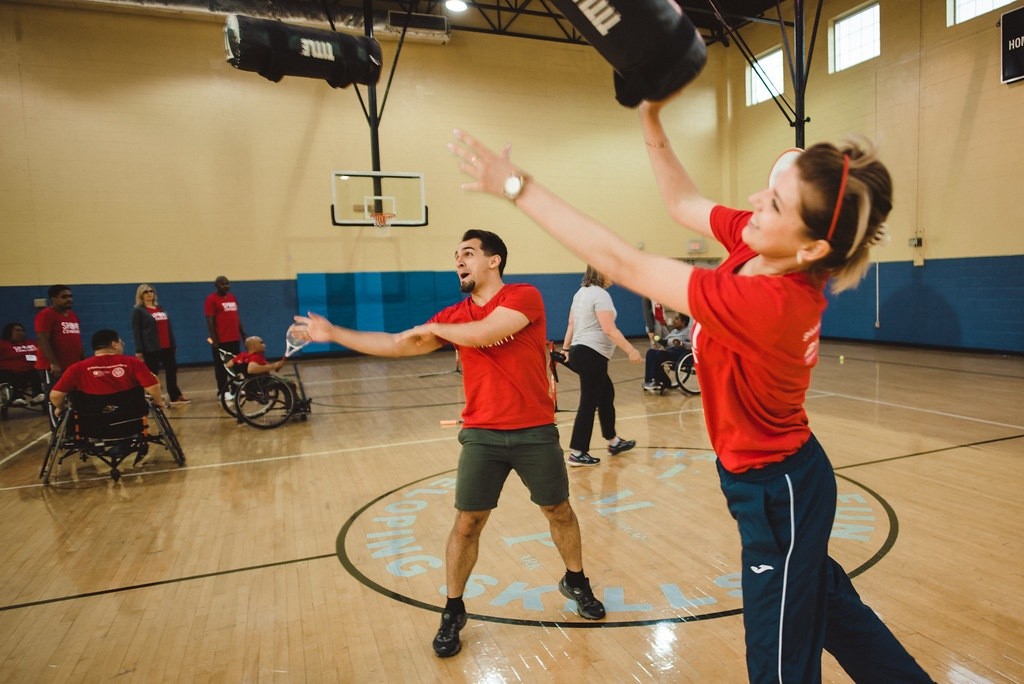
[643,138,670,149]
[561,348,570,351]
[272,363,275,369]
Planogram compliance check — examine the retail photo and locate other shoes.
[32,388,45,402]
[287,397,312,415]
[169,395,191,403]
[218,391,235,400]
[12,393,27,404]
[642,378,663,389]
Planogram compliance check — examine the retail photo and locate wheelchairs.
[0,367,53,418]
[219,363,313,430]
[38,391,187,485]
[658,352,703,395]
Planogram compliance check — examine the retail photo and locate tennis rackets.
[207,337,237,377]
[274,322,311,372]
[440,421,463,425]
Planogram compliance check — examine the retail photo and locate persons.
[131,284,194,410]
[204,276,249,402]
[291,228,607,658]
[34,285,83,445]
[226,335,313,413]
[642,295,691,394]
[0,323,49,405]
[49,330,166,439]
[560,263,638,466]
[447,86,941,684]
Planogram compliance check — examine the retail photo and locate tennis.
[654,336,660,341]
[840,360,844,364]
[840,355,844,360]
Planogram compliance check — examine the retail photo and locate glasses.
[141,289,154,293]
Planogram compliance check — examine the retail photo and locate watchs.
[502,171,532,205]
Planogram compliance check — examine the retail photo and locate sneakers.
[567,450,600,466]
[432,604,468,657]
[558,573,606,619]
[608,436,635,454]
